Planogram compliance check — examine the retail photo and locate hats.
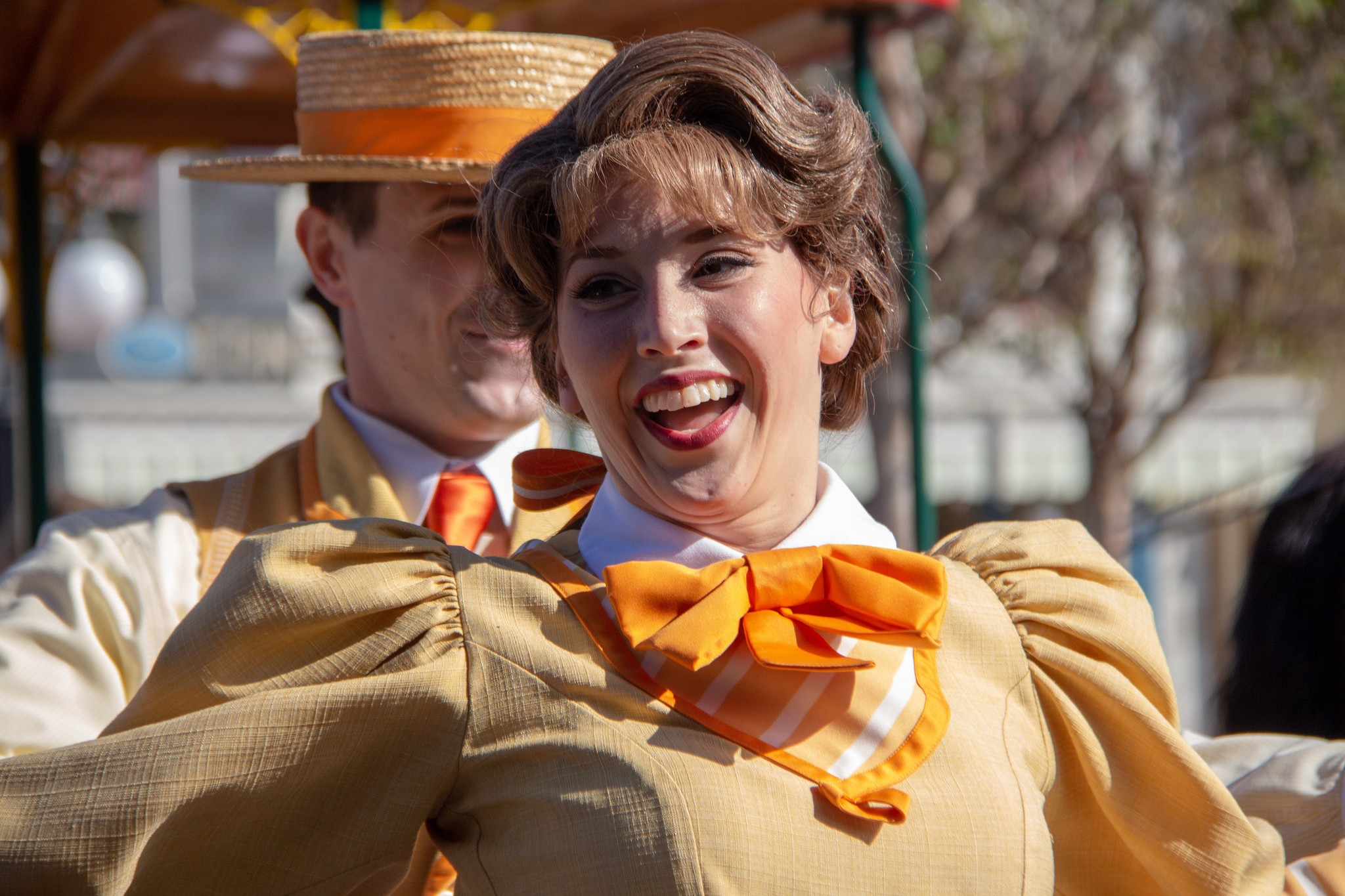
[176,29,620,186]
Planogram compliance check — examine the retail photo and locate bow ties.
[602,541,948,674]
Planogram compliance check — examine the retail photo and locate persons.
[1,33,1295,894]
[1,33,1345,893]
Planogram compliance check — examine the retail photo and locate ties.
[423,471,495,896]
